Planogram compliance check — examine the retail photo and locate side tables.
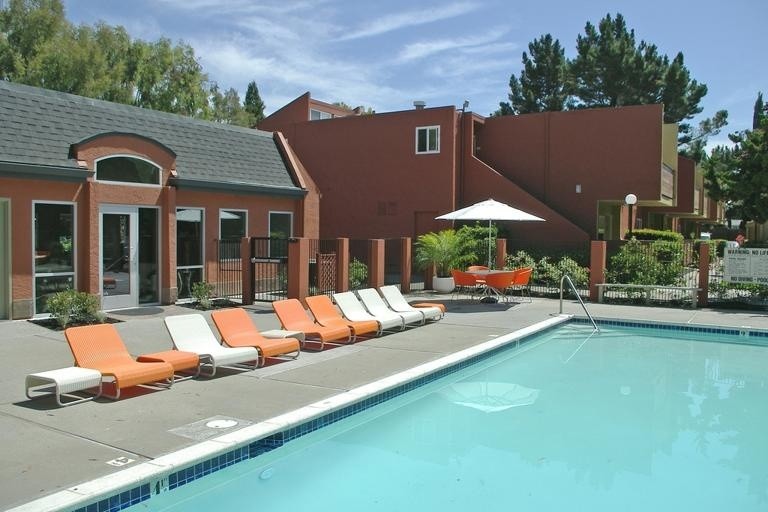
[25,366,104,407]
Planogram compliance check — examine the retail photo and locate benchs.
[595,283,703,312]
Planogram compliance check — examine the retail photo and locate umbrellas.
[439,370,543,413]
[432,198,546,268]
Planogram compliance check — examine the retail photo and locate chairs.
[164,314,260,378]
[211,308,299,365]
[357,288,424,331]
[273,299,351,351]
[379,285,441,326]
[451,258,535,302]
[304,295,380,344]
[333,292,403,337]
[65,322,175,399]
[103,278,116,289]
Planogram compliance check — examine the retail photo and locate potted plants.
[412,225,478,297]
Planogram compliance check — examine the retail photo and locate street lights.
[625,194,638,237]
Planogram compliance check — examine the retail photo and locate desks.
[413,302,445,317]
[177,265,204,303]
[259,330,305,352]
[139,350,201,387]
